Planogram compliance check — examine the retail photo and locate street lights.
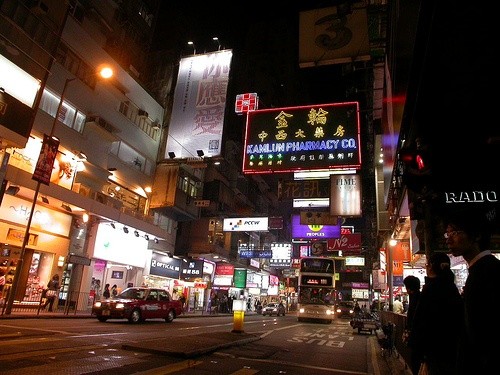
[5,66,114,316]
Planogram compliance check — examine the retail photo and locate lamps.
[145,235,149,241]
[168,151,176,159]
[110,221,115,229]
[41,196,49,204]
[197,150,204,156]
[154,238,158,243]
[134,230,139,237]
[188,40,196,55]
[60,202,73,212]
[124,226,128,233]
[213,36,220,51]
[5,183,20,196]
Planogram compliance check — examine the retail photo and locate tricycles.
[357,314,373,335]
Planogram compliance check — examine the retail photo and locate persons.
[209,293,282,313]
[41,274,59,312]
[95,280,101,300]
[172,290,178,300]
[402,276,421,375]
[354,303,367,318]
[443,209,500,375]
[103,284,110,299]
[112,284,117,296]
[411,252,463,375]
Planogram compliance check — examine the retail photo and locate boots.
[48,304,53,312]
[41,303,48,310]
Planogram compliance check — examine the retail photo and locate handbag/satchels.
[42,289,47,298]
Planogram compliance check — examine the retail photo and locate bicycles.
[380,320,397,364]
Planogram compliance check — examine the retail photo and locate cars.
[93,287,183,325]
[261,302,286,316]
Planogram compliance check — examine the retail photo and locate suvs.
[336,300,355,319]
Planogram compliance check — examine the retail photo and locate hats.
[429,253,450,263]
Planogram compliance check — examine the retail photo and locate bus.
[295,255,336,324]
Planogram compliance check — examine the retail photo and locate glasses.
[425,263,430,267]
[444,231,463,239]
